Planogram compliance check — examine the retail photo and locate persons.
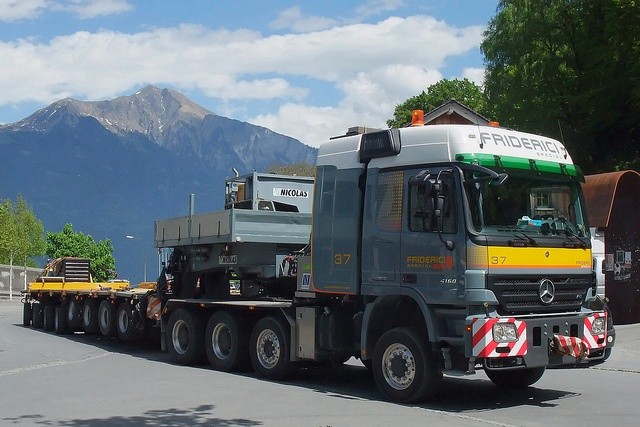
[504,192,542,227]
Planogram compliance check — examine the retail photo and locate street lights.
[126,236,146,282]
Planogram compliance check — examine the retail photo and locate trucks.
[20,110,615,403]
